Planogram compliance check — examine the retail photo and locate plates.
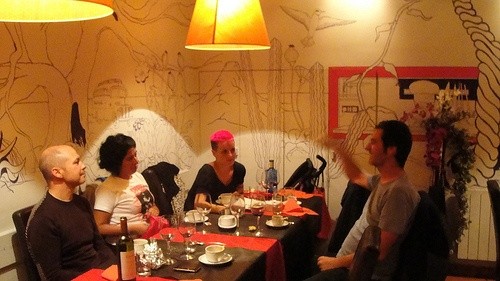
[184,216,209,223]
[198,254,233,265]
[265,220,289,227]
[283,201,301,206]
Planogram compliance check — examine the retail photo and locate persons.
[25,145,119,281]
[183,129,246,216]
[308,120,415,281]
[93,133,160,255]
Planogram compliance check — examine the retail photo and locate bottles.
[264,160,278,193]
[117,217,136,281]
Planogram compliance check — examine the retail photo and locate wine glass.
[230,194,246,236]
[176,211,196,260]
[160,214,176,264]
[195,193,212,234]
[251,192,266,237]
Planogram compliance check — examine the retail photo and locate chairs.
[12,162,382,281]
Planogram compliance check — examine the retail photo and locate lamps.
[184,0,271,51]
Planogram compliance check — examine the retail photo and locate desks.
[70,185,325,281]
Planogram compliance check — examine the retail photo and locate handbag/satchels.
[312,170,334,238]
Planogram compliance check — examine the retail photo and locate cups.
[284,187,295,201]
[205,246,225,262]
[218,215,237,228]
[218,192,233,204]
[135,251,151,277]
[133,239,148,255]
[272,215,284,226]
[272,194,282,216]
[287,196,297,202]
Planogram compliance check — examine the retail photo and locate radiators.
[457,187,496,266]
